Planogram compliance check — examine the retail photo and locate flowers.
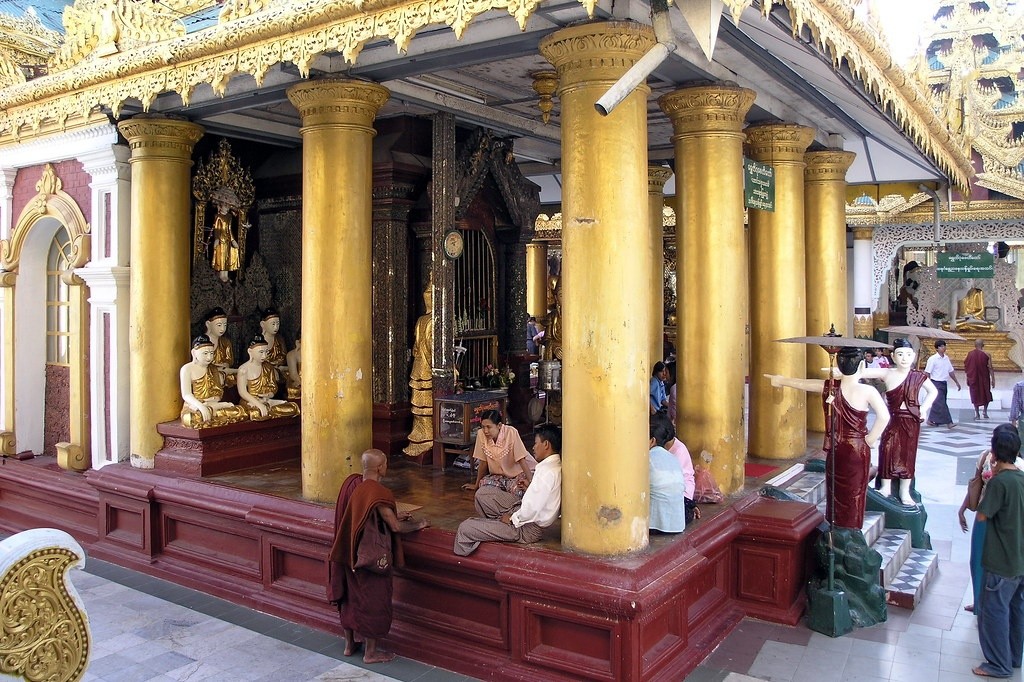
[484,356,515,388]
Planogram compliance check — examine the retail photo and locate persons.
[462,410,540,498]
[923,340,961,428]
[180,334,247,429]
[649,360,669,415]
[237,332,300,421]
[649,413,702,523]
[453,424,562,556]
[285,328,302,400]
[664,360,676,436]
[204,205,240,272]
[203,306,238,388]
[959,423,1023,615]
[861,347,891,367]
[941,278,996,332]
[649,424,687,534]
[1009,380,1023,457]
[971,430,1024,678]
[326,448,432,664]
[259,306,289,381]
[821,338,940,507]
[763,348,892,529]
[526,316,539,356]
[964,338,997,420]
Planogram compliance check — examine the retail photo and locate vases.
[492,386,512,425]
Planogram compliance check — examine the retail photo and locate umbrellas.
[878,318,969,373]
[773,324,895,592]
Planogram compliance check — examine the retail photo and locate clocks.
[441,228,465,261]
